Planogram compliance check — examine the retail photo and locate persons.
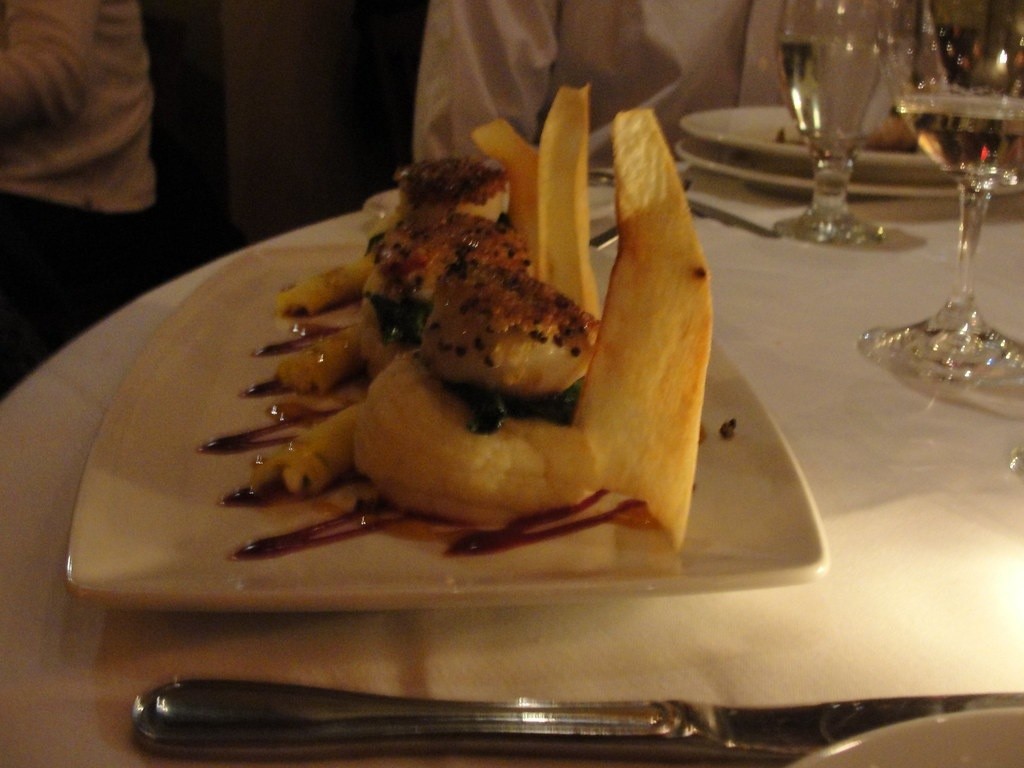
[0,0,162,405]
[413,0,1024,176]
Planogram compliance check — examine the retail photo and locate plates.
[362,184,616,224]
[675,137,1024,198]
[679,105,1009,181]
[785,706,1024,768]
[65,244,823,614]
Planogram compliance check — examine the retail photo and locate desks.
[0,160,1024,768]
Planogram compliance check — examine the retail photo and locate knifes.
[589,171,779,240]
[133,677,1024,755]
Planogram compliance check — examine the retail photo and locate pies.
[376,158,601,392]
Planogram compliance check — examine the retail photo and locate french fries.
[482,80,715,553]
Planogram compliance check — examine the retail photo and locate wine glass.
[769,0,887,250]
[873,1,1024,385]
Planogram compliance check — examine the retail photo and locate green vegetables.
[363,232,579,434]
